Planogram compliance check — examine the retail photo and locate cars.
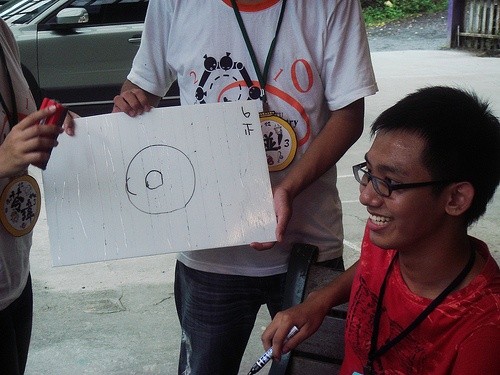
[1,0,181,117]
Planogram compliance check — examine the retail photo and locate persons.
[261,86,500,375]
[113,0,379,375]
[0,15,84,375]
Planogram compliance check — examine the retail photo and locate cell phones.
[31,96,68,170]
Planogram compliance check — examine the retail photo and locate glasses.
[352,162,447,197]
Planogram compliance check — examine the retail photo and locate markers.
[247,326,298,375]
